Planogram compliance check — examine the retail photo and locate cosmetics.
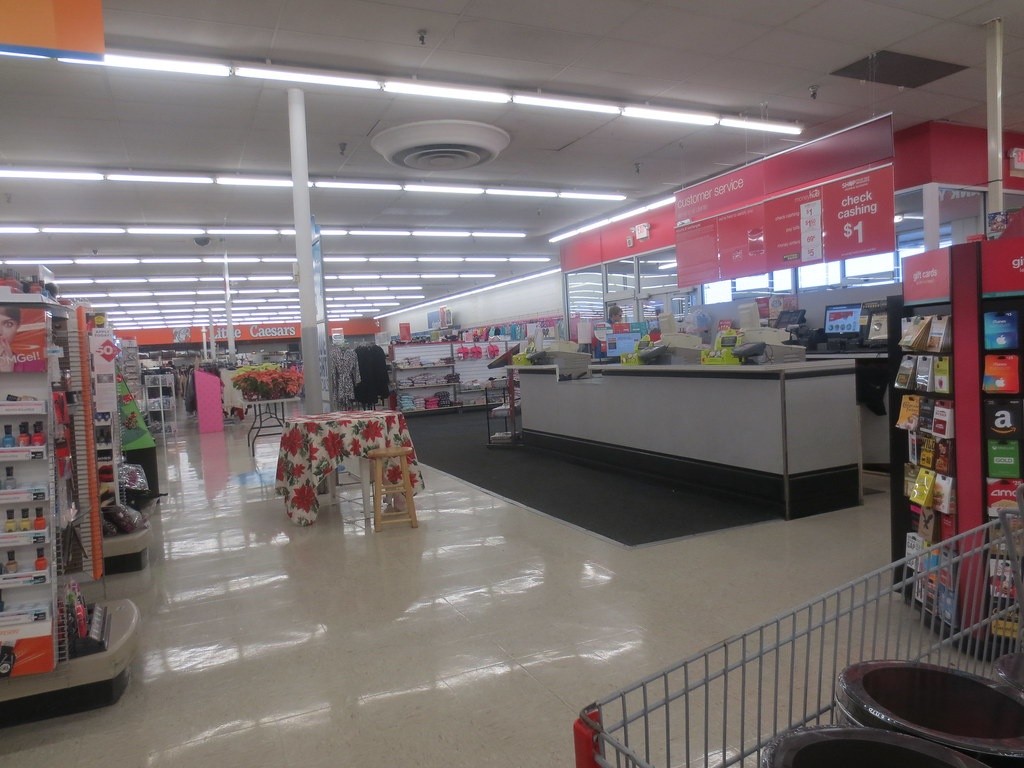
[3,419,45,447]
[7,393,40,403]
[34,507,47,531]
[2,267,55,297]
[34,547,48,572]
[5,550,19,575]
[2,465,17,490]
[4,509,18,534]
[19,510,33,533]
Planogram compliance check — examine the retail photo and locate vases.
[242,385,295,402]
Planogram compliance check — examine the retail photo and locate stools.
[367,447,419,532]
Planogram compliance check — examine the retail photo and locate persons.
[595,306,624,358]
[0,305,45,372]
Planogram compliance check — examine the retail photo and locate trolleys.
[573,482,1024,767]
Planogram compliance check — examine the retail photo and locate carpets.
[402,408,888,551]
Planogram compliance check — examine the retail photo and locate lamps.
[1006,147,1024,171]
[629,223,651,240]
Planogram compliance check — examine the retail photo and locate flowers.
[231,361,304,398]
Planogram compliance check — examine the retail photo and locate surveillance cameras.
[194,238,211,247]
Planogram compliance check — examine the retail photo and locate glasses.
[615,313,622,317]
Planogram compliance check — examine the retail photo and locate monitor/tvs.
[773,310,806,330]
[824,303,862,334]
[737,302,759,329]
[658,312,677,336]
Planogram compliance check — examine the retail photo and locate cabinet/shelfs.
[0,286,140,729]
[88,331,177,577]
[387,337,561,418]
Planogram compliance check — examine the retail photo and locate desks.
[284,410,402,519]
[238,396,301,457]
